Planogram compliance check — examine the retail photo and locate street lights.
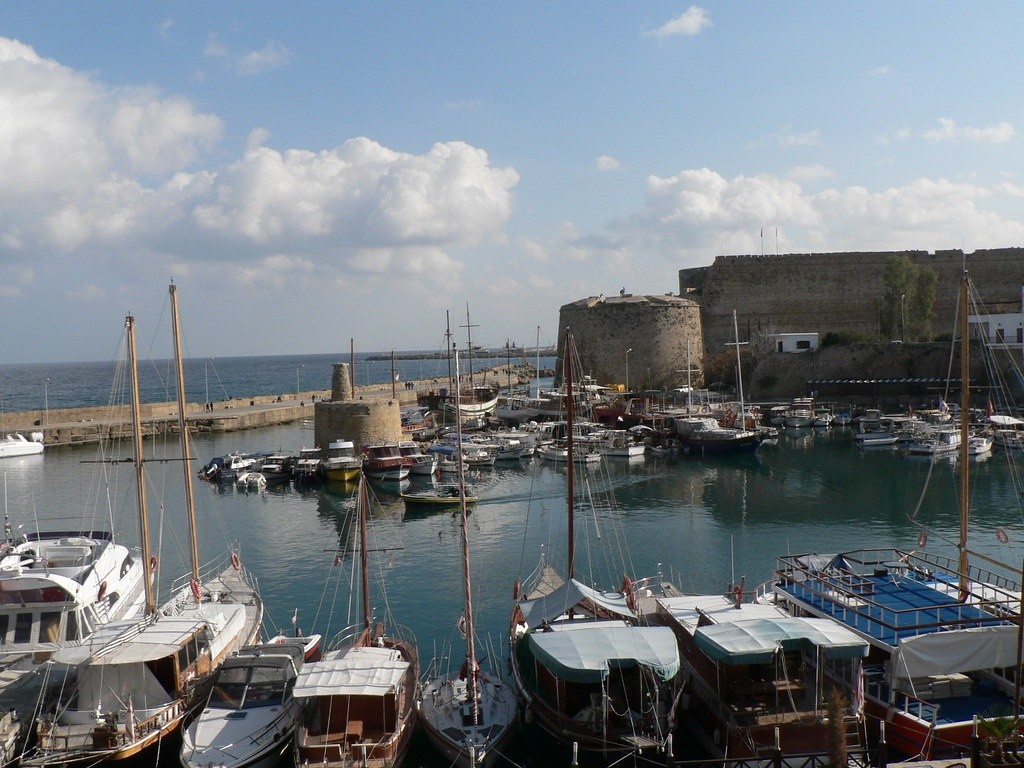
[900,293,906,343]
[624,349,633,393]
[205,357,214,402]
[44,376,51,426]
[877,295,883,339]
[366,361,374,385]
[296,365,305,393]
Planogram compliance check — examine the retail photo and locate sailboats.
[0,266,1024,767]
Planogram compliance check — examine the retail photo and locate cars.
[673,385,694,393]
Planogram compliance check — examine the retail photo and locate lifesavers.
[460,661,480,683]
[623,576,633,596]
[98,581,107,602]
[460,620,467,635]
[190,578,201,599]
[231,553,241,571]
[734,586,742,602]
[513,581,520,600]
[150,556,158,575]
[44,588,63,602]
[996,528,1009,544]
[918,534,927,547]
